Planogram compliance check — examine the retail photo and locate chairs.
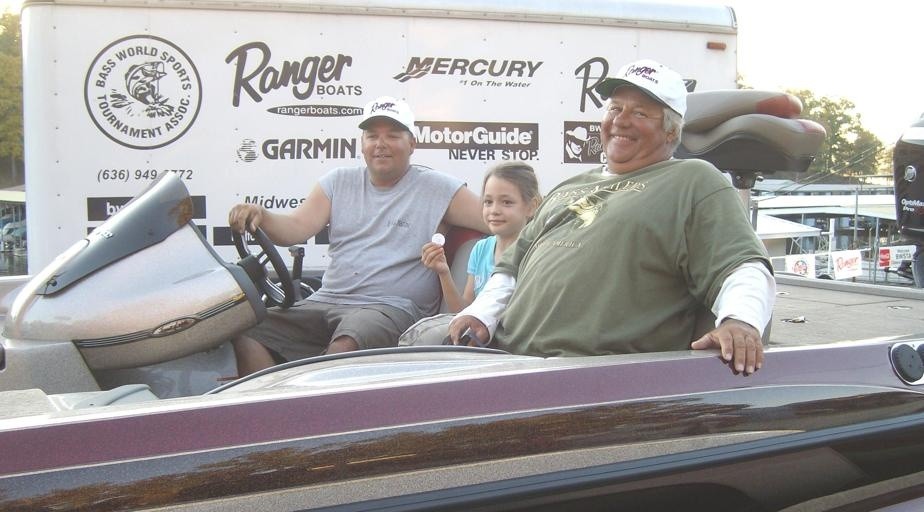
[439,231,487,314]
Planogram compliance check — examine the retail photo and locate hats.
[596,60,688,118]
[357,98,418,144]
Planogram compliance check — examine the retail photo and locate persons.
[442,58,776,375]
[227,96,495,382]
[397,159,541,348]
